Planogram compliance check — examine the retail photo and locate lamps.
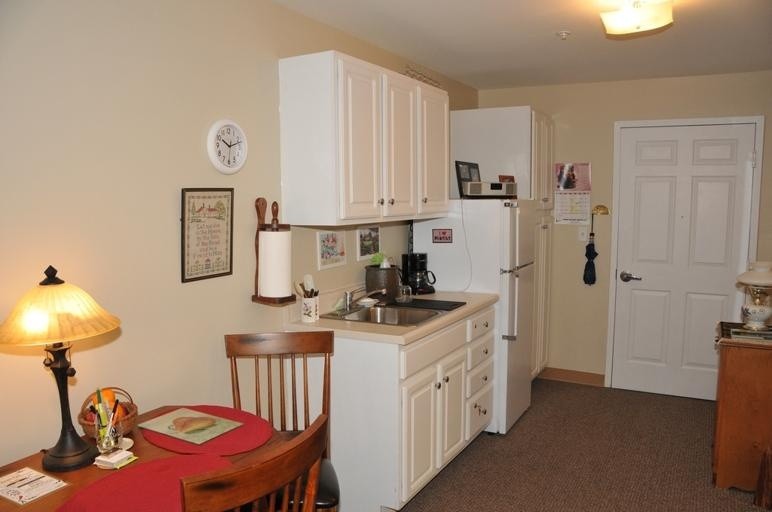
[1,264,121,474]
[737,259,771,332]
[178,330,340,512]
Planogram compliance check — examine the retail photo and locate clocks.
[208,119,249,176]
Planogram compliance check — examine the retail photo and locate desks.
[0,405,294,512]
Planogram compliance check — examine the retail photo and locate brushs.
[403,252,436,293]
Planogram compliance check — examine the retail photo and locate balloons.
[208,119,249,176]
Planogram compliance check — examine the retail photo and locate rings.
[300,282,320,298]
[90,389,119,453]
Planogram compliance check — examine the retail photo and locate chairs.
[178,330,340,512]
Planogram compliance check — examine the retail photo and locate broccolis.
[413,199,532,434]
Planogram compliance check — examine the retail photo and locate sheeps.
[344,287,387,312]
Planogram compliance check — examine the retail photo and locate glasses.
[1,264,121,474]
[737,259,771,332]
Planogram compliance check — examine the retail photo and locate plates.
[451,104,555,210]
[277,51,417,230]
[711,322,772,491]
[416,79,451,223]
[531,216,554,382]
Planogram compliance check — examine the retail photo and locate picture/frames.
[0,405,294,512]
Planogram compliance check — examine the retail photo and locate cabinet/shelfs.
[711,322,772,491]
[277,51,417,230]
[531,216,554,382]
[286,320,467,508]
[451,104,555,210]
[468,306,493,442]
[416,79,451,223]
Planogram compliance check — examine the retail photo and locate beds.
[583,213,598,286]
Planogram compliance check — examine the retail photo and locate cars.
[92,422,124,454]
